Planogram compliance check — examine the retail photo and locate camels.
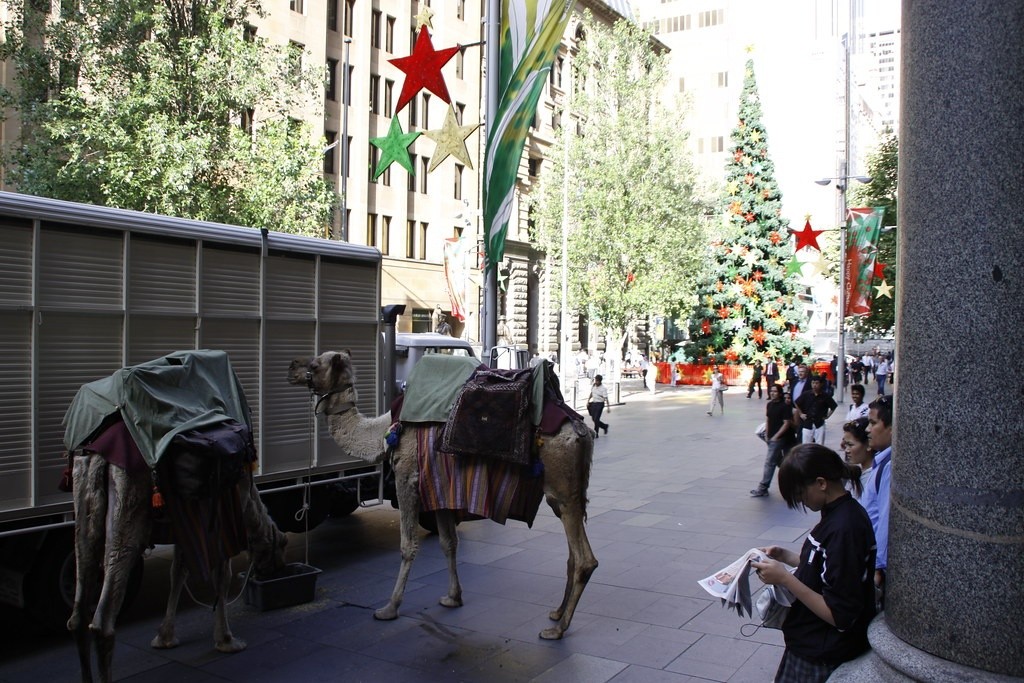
[62,412,289,683]
[287,350,598,642]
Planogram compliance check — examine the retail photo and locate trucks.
[0,186,480,637]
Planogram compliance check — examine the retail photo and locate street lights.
[813,175,873,405]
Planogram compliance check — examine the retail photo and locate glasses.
[876,394,887,404]
[850,420,860,428]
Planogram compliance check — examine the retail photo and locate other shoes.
[604,424,608,435]
[750,488,768,496]
[595,433,599,438]
[707,411,713,416]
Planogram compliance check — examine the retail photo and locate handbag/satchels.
[587,402,594,416]
[756,424,767,442]
[755,588,788,629]
[719,383,728,391]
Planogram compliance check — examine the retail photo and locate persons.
[640,354,658,394]
[747,443,878,683]
[747,358,763,399]
[782,352,838,455]
[624,350,633,368]
[587,375,610,436]
[847,384,872,422]
[706,364,725,415]
[842,394,894,615]
[764,356,779,400]
[829,344,894,397]
[670,357,681,387]
[750,384,794,498]
[437,312,453,356]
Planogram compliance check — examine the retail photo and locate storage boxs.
[239,561,318,611]
